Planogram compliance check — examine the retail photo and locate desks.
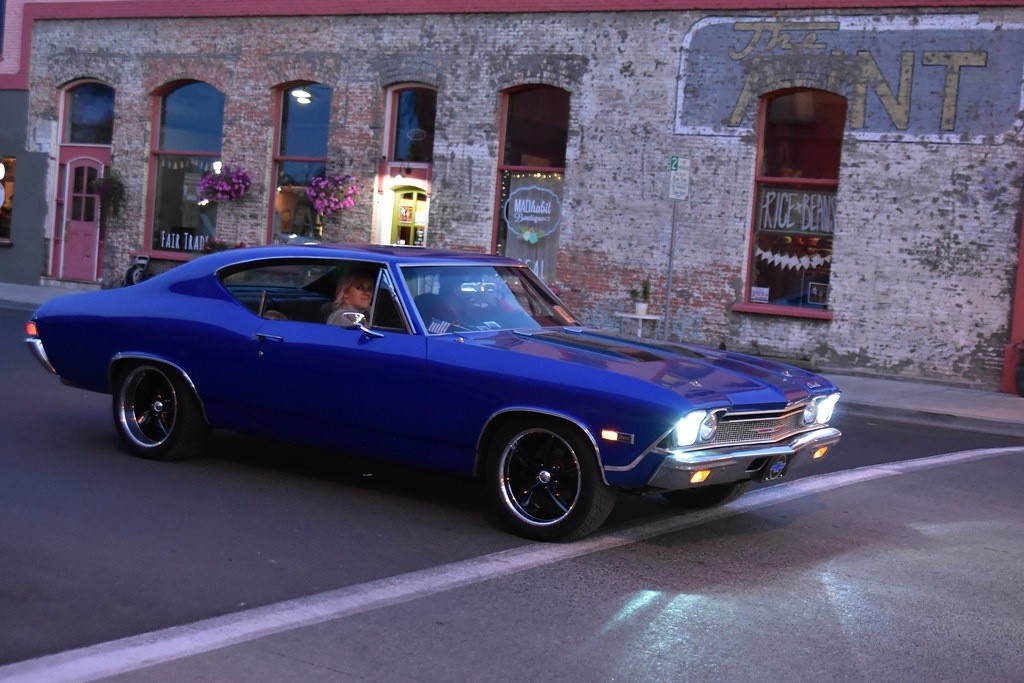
[614,312,665,340]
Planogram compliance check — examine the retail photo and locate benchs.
[241,292,336,325]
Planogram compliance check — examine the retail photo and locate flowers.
[305,176,365,216]
[86,176,124,217]
[198,170,252,203]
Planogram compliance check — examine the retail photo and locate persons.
[327,270,469,327]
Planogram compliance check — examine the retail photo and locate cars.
[27,244,845,546]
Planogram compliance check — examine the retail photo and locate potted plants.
[628,279,653,316]
